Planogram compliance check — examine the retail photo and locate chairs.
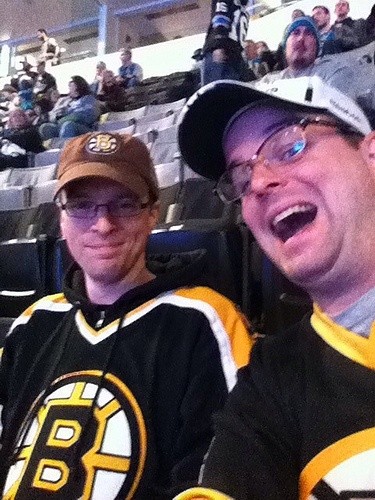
[0,71,374,353]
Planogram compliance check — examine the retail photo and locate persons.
[164,0,375,98]
[0,131,254,500]
[175,75,375,500]
[0,28,144,170]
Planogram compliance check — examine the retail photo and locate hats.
[175,73,372,180]
[281,15,322,58]
[51,131,161,200]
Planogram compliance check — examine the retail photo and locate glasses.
[211,116,350,207]
[59,198,157,219]
